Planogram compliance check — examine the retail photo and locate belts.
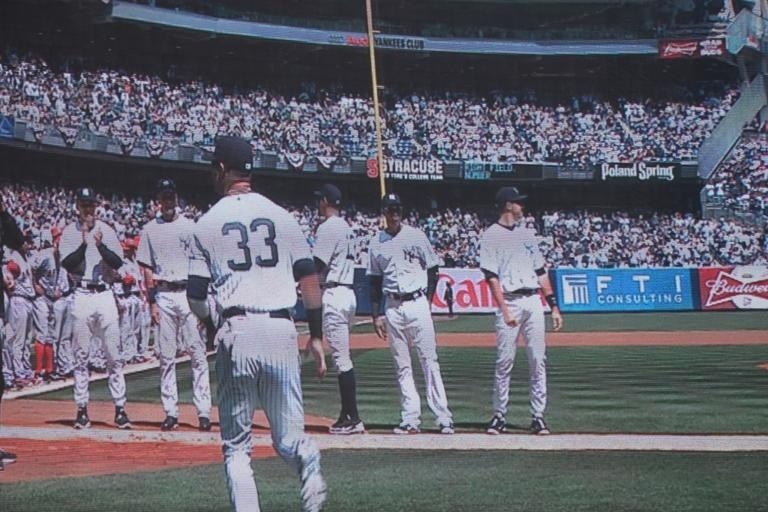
[387,291,420,301]
[218,306,291,321]
[159,280,189,291]
[75,281,107,292]
[325,281,354,290]
[512,288,539,296]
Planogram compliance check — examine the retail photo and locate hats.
[202,136,254,174]
[78,187,100,203]
[156,177,176,194]
[381,193,404,210]
[494,187,527,203]
[313,184,342,208]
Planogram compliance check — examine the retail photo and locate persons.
[185,135,327,511]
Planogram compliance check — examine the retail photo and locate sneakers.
[488,416,508,435]
[327,419,366,435]
[112,412,131,429]
[292,437,327,512]
[530,416,550,435]
[1,448,17,471]
[440,417,455,435]
[5,351,153,393]
[161,416,178,431]
[393,421,423,435]
[74,411,92,429]
[198,415,210,429]
